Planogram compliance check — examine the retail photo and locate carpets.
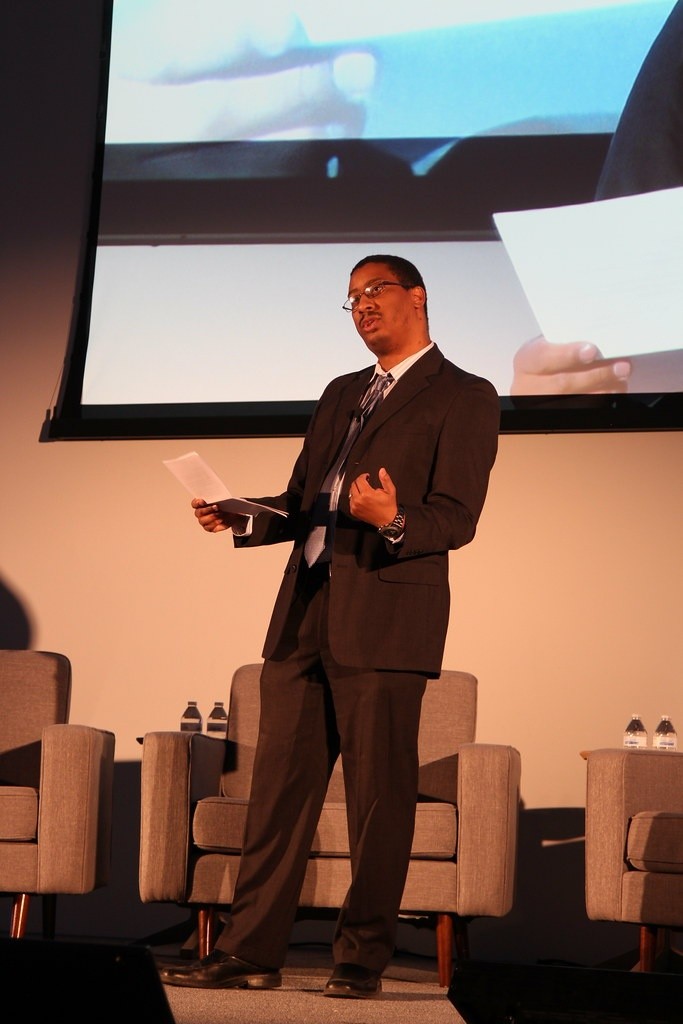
[147,945,468,1024]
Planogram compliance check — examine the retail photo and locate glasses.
[342,280,412,313]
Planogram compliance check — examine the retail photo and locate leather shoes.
[324,963,382,999]
[160,949,282,988]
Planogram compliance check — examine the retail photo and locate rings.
[347,494,353,499]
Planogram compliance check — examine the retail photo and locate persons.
[511,1,683,395]
[156,252,503,999]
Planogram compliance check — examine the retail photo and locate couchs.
[139,663,522,988]
[0,650,116,938]
[578,748,683,973]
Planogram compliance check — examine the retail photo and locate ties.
[304,375,394,568]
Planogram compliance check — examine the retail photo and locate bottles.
[653,715,678,751]
[205,701,229,740]
[180,701,203,732]
[624,713,648,749]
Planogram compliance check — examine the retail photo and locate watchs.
[379,505,406,539]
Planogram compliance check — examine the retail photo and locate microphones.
[355,406,364,418]
